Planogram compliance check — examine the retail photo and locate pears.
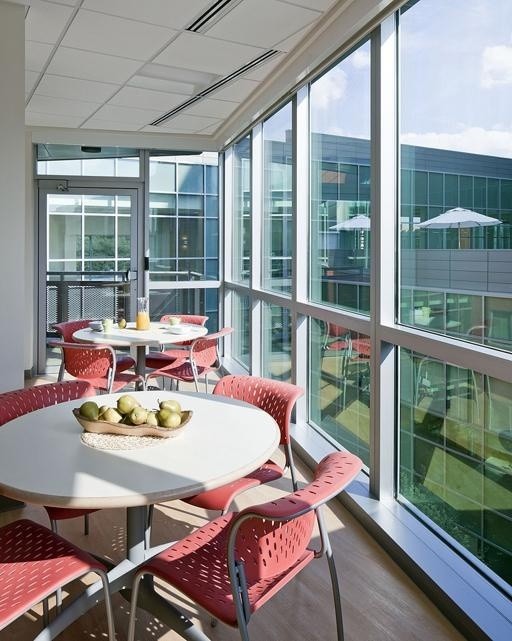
[80,395,182,429]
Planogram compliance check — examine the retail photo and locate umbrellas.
[417,206,503,249]
[328,215,371,268]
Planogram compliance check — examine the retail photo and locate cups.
[169,316,181,326]
[102,319,113,332]
[136,296,151,330]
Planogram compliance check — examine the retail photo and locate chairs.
[145,315,209,391]
[146,327,233,394]
[51,319,137,373]
[0,379,102,536]
[47,342,145,394]
[127,451,365,641]
[414,319,490,405]
[321,320,370,412]
[0,519,116,641]
[148,374,304,536]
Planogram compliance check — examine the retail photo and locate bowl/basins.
[89,321,102,330]
[170,325,192,335]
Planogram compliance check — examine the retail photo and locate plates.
[72,407,192,437]
